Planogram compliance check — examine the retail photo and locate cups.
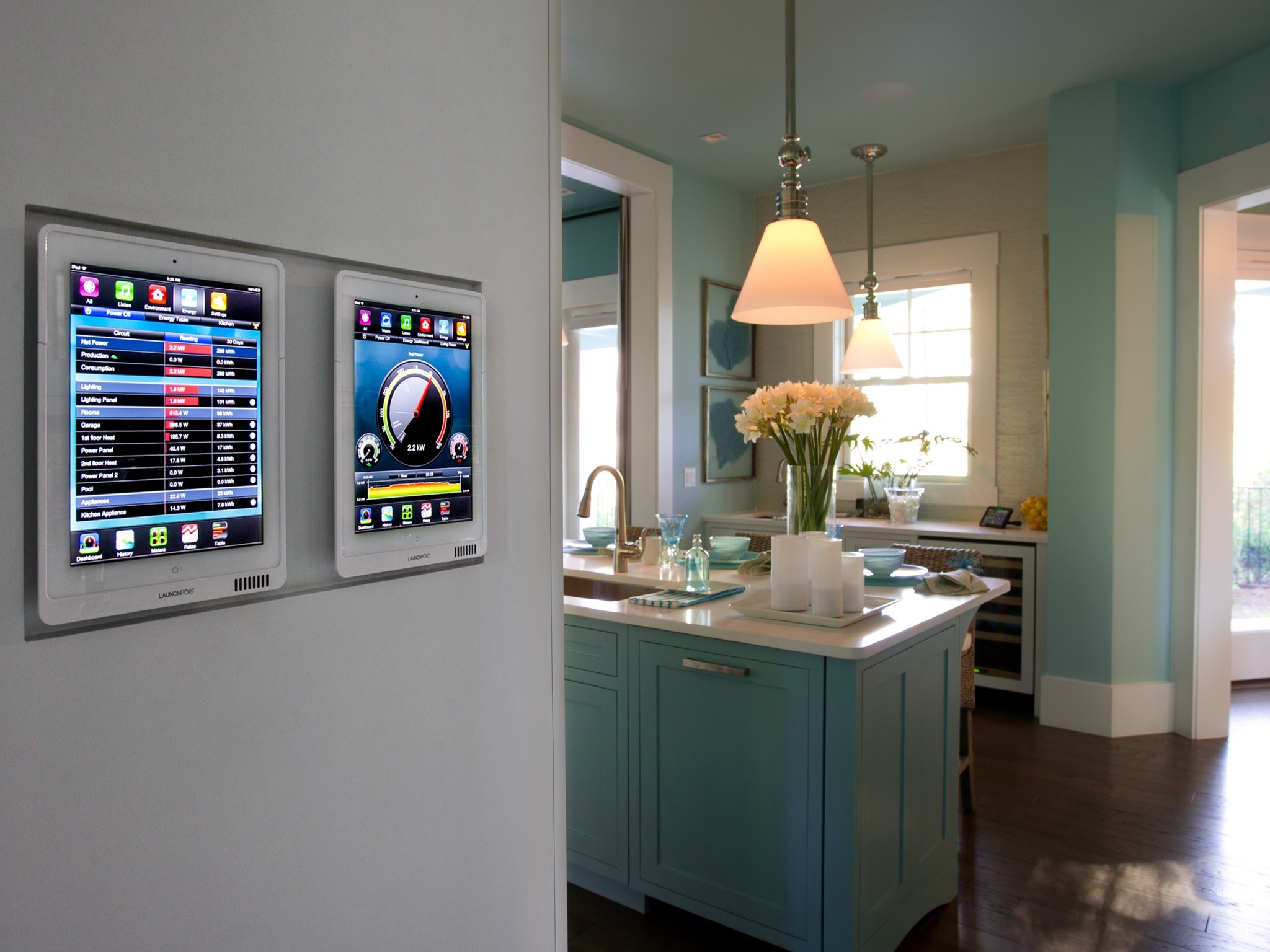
[634,536,660,565]
[769,531,865,618]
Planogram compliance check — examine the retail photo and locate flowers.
[733,380,875,531]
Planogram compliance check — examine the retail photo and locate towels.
[912,568,991,595]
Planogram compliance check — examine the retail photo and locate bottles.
[684,533,710,593]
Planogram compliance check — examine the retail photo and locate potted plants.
[839,431,978,519]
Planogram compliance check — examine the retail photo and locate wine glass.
[655,513,690,569]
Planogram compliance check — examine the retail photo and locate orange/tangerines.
[1020,495,1048,530]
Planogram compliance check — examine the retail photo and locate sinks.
[562,567,666,602]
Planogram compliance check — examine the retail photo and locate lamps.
[730,0,854,325]
[839,144,904,374]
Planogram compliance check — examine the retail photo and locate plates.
[704,550,761,564]
[863,563,930,580]
[565,541,616,552]
[728,589,899,629]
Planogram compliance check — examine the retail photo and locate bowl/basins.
[857,546,907,577]
[708,535,751,561]
[583,527,617,548]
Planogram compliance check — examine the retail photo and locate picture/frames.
[701,279,757,381]
[703,385,757,482]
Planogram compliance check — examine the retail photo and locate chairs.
[890,543,982,811]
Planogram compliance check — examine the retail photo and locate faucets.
[577,463,648,574]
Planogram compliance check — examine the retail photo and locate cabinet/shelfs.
[840,532,1035,702]
[563,604,983,952]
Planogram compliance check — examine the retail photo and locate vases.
[786,465,838,535]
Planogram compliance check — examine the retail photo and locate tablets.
[979,506,1012,529]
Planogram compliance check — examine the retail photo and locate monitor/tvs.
[33,222,300,624]
[333,271,486,579]
